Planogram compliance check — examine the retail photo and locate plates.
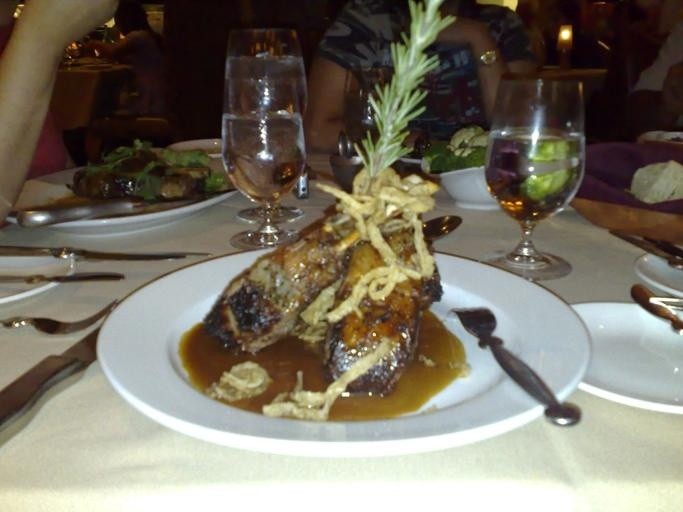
[0,256,76,307]
[569,301,683,418]
[96,245,593,455]
[7,137,237,239]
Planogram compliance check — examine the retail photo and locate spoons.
[609,228,682,271]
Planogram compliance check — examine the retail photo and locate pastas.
[262,184,435,421]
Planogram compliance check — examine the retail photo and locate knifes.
[2,271,126,283]
[630,282,683,338]
[0,328,102,430]
[0,245,185,262]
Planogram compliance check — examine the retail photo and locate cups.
[343,68,385,157]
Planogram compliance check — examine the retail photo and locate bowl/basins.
[330,154,405,194]
[420,153,502,212]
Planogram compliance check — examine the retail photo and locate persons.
[89,5,164,70]
[0,0,120,227]
[627,19,683,130]
[305,0,511,155]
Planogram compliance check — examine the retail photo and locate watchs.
[475,48,503,67]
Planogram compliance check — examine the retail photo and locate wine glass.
[219,27,307,250]
[479,75,587,282]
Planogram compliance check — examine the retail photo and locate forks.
[447,303,580,430]
[0,299,116,335]
